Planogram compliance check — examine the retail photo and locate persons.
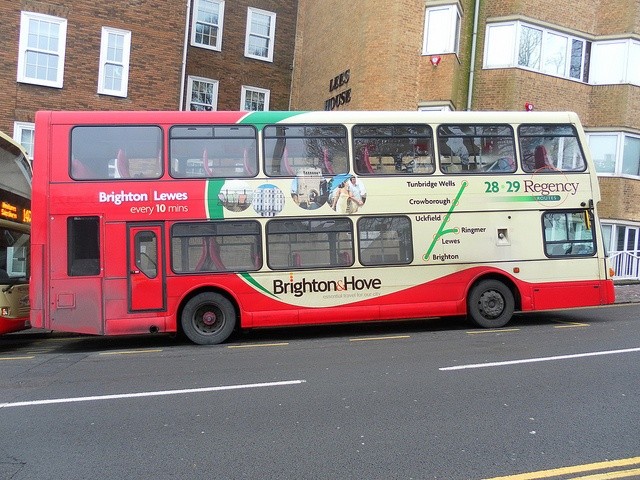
[346,176,363,213]
[330,182,345,210]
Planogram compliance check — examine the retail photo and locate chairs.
[209,236,227,270]
[500,156,515,172]
[115,149,130,178]
[338,252,344,264]
[201,148,215,176]
[250,246,260,270]
[342,252,349,264]
[533,145,555,170]
[292,254,304,266]
[355,147,373,173]
[370,255,382,263]
[242,147,255,176]
[282,146,296,175]
[73,159,84,179]
[192,238,209,271]
[319,145,335,176]
[384,254,398,261]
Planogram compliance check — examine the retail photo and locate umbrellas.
[328,174,353,194]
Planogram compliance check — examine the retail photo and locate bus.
[29,109,616,344]
[1,130,32,335]
